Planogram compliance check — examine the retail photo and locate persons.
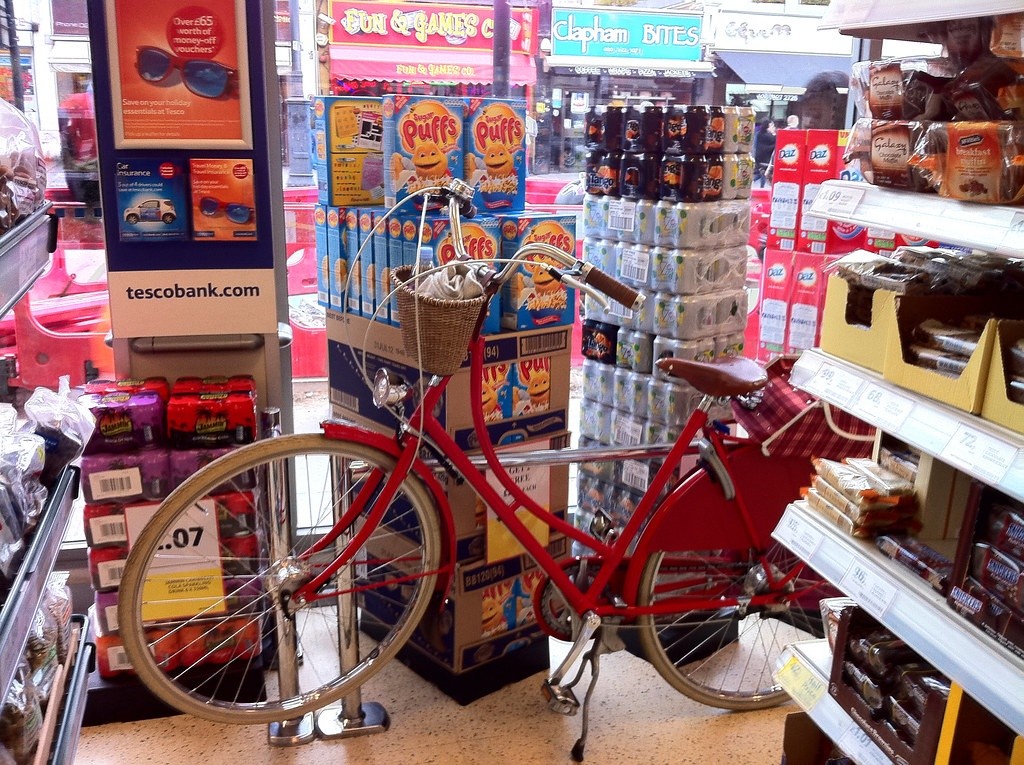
[753,118,776,188]
[55,106,87,202]
[784,115,801,130]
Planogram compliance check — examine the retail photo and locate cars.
[124,199,176,225]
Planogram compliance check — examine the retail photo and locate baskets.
[391,267,488,377]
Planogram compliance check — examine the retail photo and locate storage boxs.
[756,125,937,364]
[312,94,575,674]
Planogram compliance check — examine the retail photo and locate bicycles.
[117,174,851,762]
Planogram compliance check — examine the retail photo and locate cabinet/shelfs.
[765,0,1024,764]
[0,198,97,765]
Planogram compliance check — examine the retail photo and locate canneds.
[573,103,755,557]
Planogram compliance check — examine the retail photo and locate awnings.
[712,50,852,95]
[328,45,537,86]
[48,37,292,75]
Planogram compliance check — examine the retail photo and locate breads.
[804,453,923,541]
[855,12,1024,212]
